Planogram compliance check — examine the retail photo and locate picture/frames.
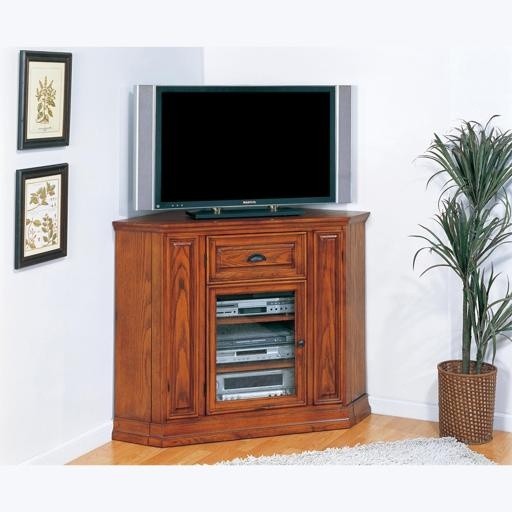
[16,51,68,269]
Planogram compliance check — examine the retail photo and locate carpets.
[207,436,495,464]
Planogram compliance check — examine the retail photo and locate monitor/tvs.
[135,84,353,220]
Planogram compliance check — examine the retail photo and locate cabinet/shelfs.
[111,208,373,443]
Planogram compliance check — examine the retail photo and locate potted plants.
[407,114,509,445]
